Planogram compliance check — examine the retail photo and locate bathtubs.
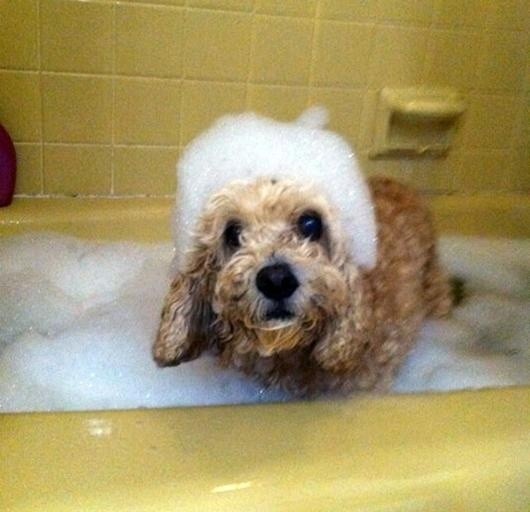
[0,195,530,512]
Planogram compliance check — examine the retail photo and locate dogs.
[152,172,470,400]
